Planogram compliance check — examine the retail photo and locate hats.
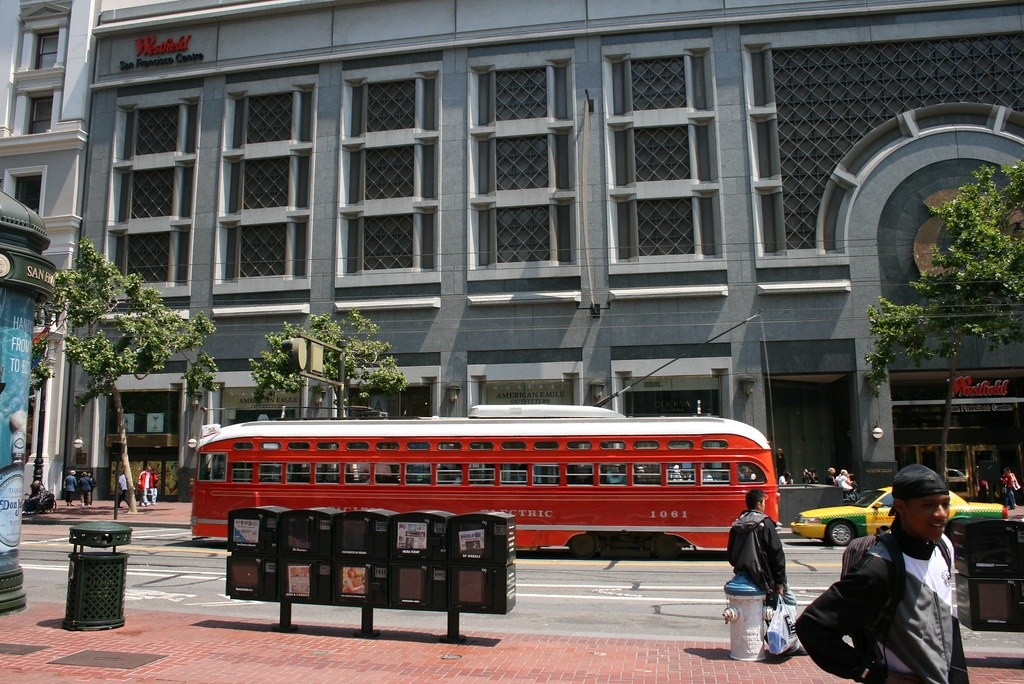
[81,472,88,476]
[69,470,76,475]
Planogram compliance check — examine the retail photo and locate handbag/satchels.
[64,478,66,489]
[766,593,800,656]
[841,479,853,491]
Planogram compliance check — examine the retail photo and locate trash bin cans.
[64,521,132,632]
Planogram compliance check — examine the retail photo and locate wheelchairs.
[34,489,57,514]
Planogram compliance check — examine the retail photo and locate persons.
[64,470,77,506]
[738,466,757,480]
[726,488,809,656]
[607,464,627,484]
[972,466,1021,510]
[343,569,364,593]
[118,471,130,508]
[795,464,969,684]
[138,466,159,507]
[78,472,96,507]
[776,448,854,526]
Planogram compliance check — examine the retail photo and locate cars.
[790,487,1007,547]
[948,469,967,492]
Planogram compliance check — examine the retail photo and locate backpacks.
[840,536,952,642]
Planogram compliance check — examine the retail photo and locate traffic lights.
[111,442,125,454]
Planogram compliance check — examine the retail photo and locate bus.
[190,404,781,561]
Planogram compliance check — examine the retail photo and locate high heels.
[1008,508,1014,510]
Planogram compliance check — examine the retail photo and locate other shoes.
[139,503,145,507]
[1013,505,1017,508]
[118,506,124,508]
[67,501,93,508]
[784,646,808,656]
[146,502,151,505]
[152,503,157,506]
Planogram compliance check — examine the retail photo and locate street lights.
[33,273,64,480]
